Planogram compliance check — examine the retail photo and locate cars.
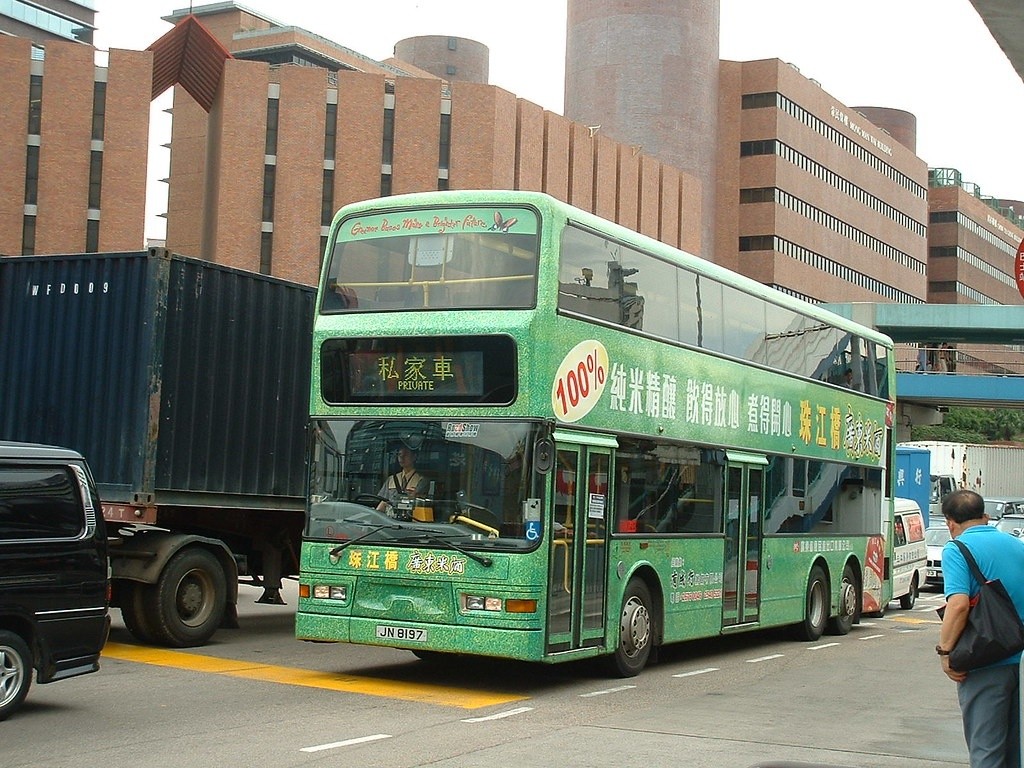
[0,441,113,720]
[926,495,1024,591]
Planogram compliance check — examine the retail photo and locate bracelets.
[936,645,954,655]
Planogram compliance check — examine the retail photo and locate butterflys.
[488,211,518,232]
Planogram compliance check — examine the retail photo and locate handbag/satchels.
[936,540,1024,672]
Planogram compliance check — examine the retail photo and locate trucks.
[0,249,326,649]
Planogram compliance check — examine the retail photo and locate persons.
[376,444,428,521]
[936,490,1024,768]
[916,342,957,375]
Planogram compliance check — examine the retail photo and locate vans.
[889,499,928,609]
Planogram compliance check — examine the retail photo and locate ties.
[402,475,407,489]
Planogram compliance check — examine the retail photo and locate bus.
[290,187,895,675]
